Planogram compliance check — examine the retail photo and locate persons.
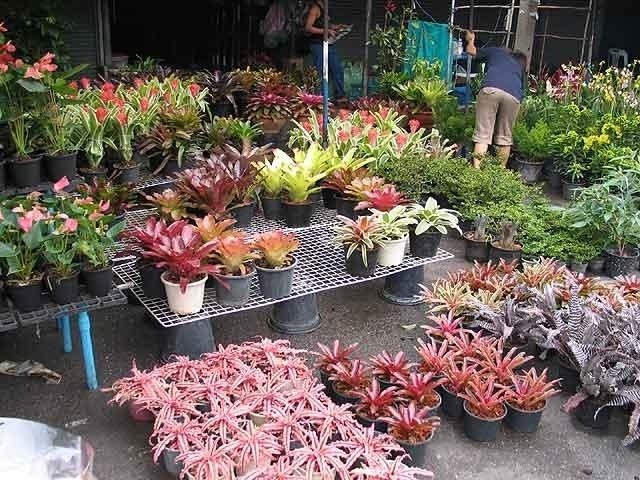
[465,29,528,170]
[304,0,350,104]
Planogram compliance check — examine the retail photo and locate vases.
[47,149,76,180]
[81,166,109,187]
[7,276,50,314]
[85,268,116,301]
[47,265,85,310]
[5,156,44,189]
[110,160,145,185]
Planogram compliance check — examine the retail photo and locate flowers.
[83,108,115,168]
[24,49,76,154]
[42,205,78,280]
[76,205,119,273]
[114,101,139,165]
[2,207,42,285]
[0,19,32,162]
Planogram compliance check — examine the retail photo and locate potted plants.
[121,340,431,480]
[563,135,590,204]
[255,229,300,298]
[400,75,442,128]
[489,222,525,268]
[507,117,547,186]
[298,92,325,141]
[306,339,367,375]
[563,337,627,432]
[260,137,285,223]
[321,359,379,407]
[427,319,526,379]
[201,67,234,115]
[406,198,463,258]
[585,241,608,276]
[347,385,399,432]
[461,215,489,263]
[117,213,168,301]
[417,260,640,444]
[379,147,567,274]
[425,350,468,419]
[369,348,419,393]
[215,229,253,308]
[375,207,415,267]
[412,339,446,383]
[152,154,235,231]
[138,124,186,177]
[281,141,335,228]
[325,152,363,219]
[399,75,446,127]
[569,245,588,274]
[245,89,285,134]
[573,166,640,279]
[333,212,376,277]
[387,405,434,471]
[143,229,227,316]
[505,368,563,435]
[396,376,443,418]
[208,140,258,226]
[456,378,506,440]
